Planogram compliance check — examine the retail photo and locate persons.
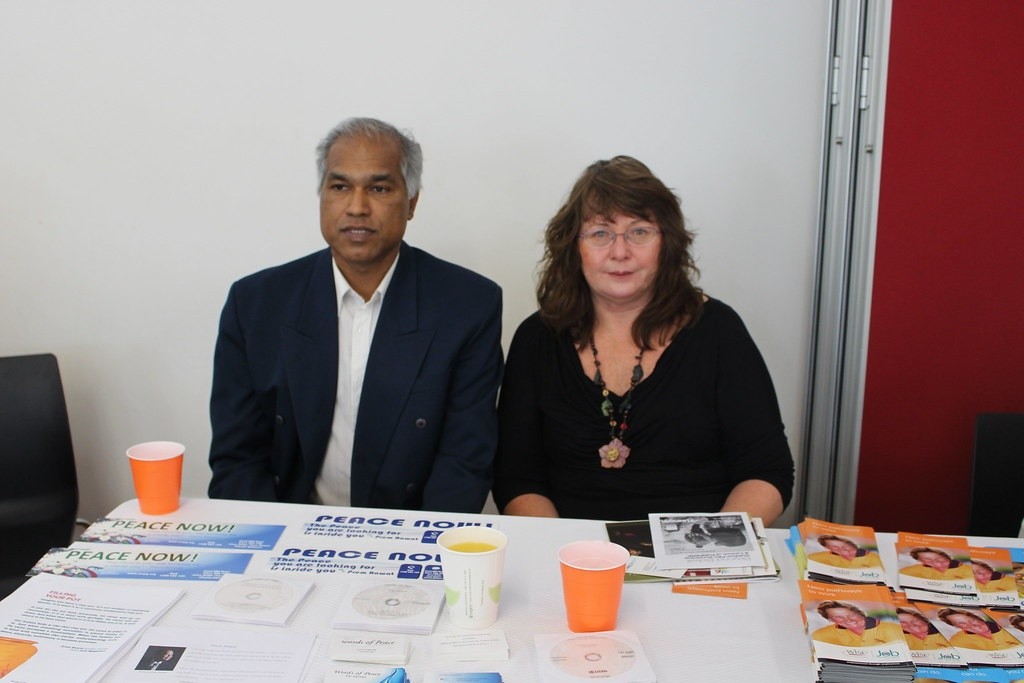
[807,534,881,569]
[208,120,505,514]
[938,607,1020,651]
[972,560,1016,593]
[895,606,952,649]
[137,648,175,670]
[811,600,904,647]
[491,155,795,529]
[899,546,973,581]
[1009,614,1024,632]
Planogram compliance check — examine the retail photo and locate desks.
[0,499,1023,682]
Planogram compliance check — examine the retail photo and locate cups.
[558,540,629,635]
[437,527,509,630]
[126,440,189,515]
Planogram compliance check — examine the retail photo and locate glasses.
[579,227,660,249]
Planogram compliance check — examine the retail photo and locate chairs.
[0,354,92,602]
[969,412,1024,539]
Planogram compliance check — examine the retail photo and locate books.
[790,518,1024,683]
[605,512,781,585]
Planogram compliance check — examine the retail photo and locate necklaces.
[588,336,646,468]
